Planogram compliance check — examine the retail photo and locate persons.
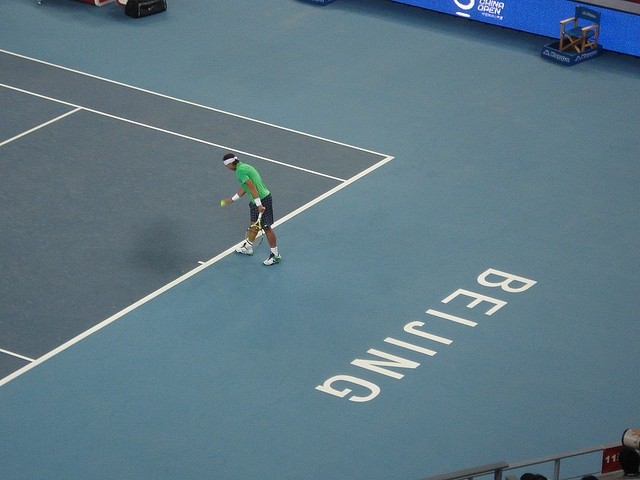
[223,153,283,266]
[616,447,640,480]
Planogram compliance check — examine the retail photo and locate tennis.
[220,200,225,206]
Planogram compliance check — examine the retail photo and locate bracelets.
[254,198,262,207]
[232,193,240,202]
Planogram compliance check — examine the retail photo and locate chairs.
[557,4,603,55]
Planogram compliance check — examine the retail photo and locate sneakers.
[235,243,254,256]
[262,252,282,266]
